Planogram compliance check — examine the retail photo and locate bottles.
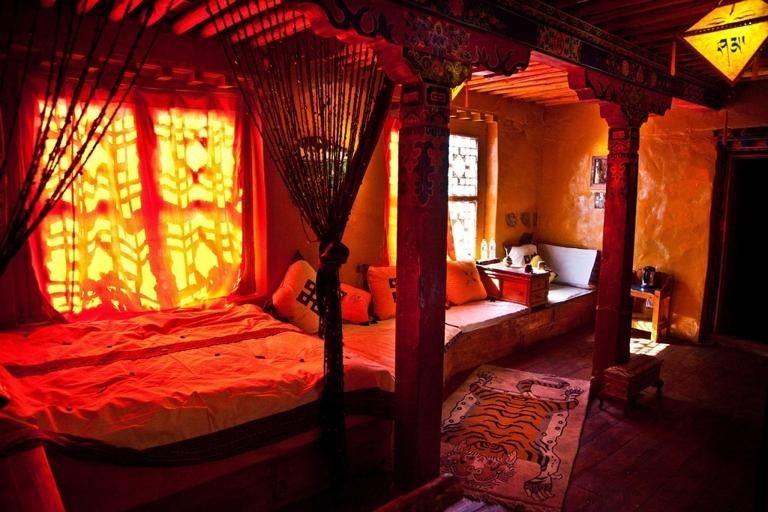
[481,239,495,260]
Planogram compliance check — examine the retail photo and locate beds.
[0,297,397,511]
[338,243,601,388]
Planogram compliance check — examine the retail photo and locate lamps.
[669,0,768,148]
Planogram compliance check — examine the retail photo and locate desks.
[477,253,551,311]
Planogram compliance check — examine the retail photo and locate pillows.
[360,264,397,320]
[448,258,484,307]
[270,250,345,333]
[339,282,372,324]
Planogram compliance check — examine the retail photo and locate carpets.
[440,361,592,512]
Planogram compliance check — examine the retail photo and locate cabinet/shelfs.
[631,267,674,342]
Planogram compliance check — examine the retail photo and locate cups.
[525,264,533,273]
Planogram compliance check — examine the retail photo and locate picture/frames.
[590,155,609,189]
[592,190,606,210]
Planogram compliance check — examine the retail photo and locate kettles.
[640,265,656,290]
